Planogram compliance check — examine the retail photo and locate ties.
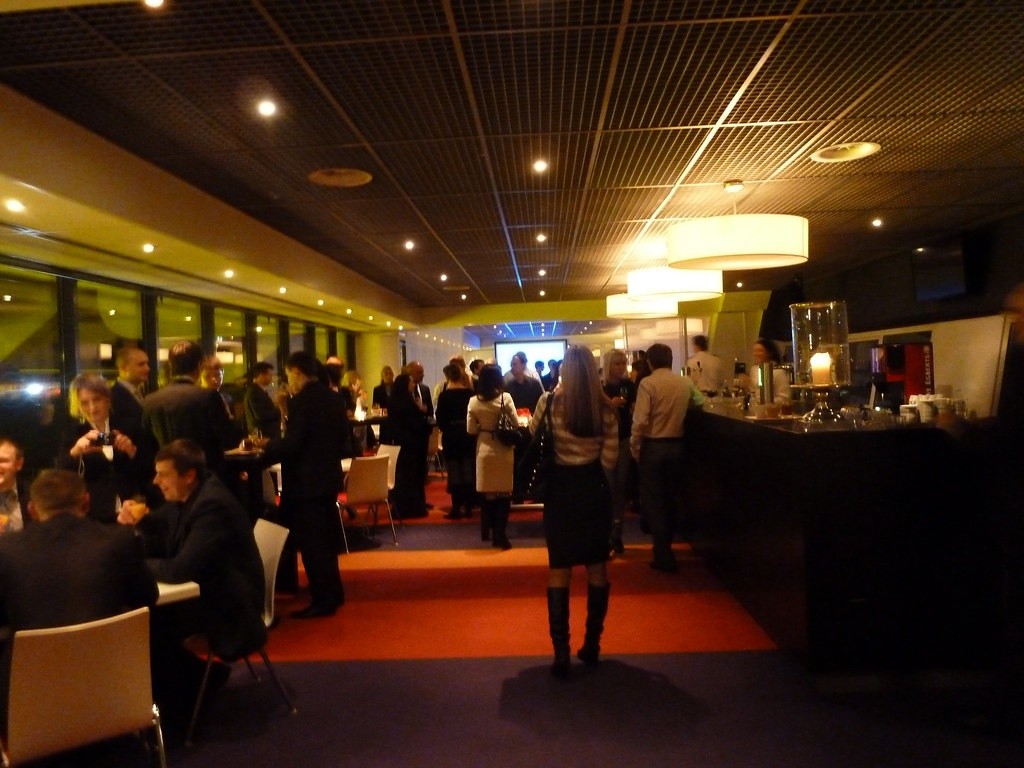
[414,385,420,400]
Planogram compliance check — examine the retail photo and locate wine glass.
[128,493,146,538]
[620,387,627,409]
[248,426,264,452]
[706,386,717,408]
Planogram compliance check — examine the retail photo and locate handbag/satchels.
[496,392,524,447]
[516,390,556,494]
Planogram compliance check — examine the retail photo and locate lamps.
[606,292,679,319]
[667,180,814,270]
[627,266,725,304]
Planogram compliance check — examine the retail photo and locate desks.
[222,448,284,503]
[156,580,200,607]
[349,416,388,452]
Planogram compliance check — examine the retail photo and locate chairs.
[177,516,298,747]
[334,458,360,533]
[336,454,399,555]
[365,444,402,537]
[427,426,446,478]
[0,607,167,767]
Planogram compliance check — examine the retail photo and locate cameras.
[90,432,116,447]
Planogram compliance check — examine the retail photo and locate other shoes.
[416,494,683,572]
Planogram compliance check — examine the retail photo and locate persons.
[196,354,239,420]
[59,371,161,523]
[684,335,732,400]
[137,340,243,492]
[130,435,268,726]
[3,468,158,739]
[529,345,620,680]
[245,362,283,442]
[109,348,150,433]
[628,342,707,571]
[0,436,42,536]
[751,337,793,402]
[467,364,521,551]
[260,350,348,620]
[327,345,652,517]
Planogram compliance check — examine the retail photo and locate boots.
[577,583,609,664]
[547,587,572,682]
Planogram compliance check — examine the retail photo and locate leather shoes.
[290,601,335,618]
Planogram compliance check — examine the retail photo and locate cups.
[937,397,953,415]
[370,403,387,417]
[918,400,936,425]
[900,405,916,425]
[243,437,255,453]
